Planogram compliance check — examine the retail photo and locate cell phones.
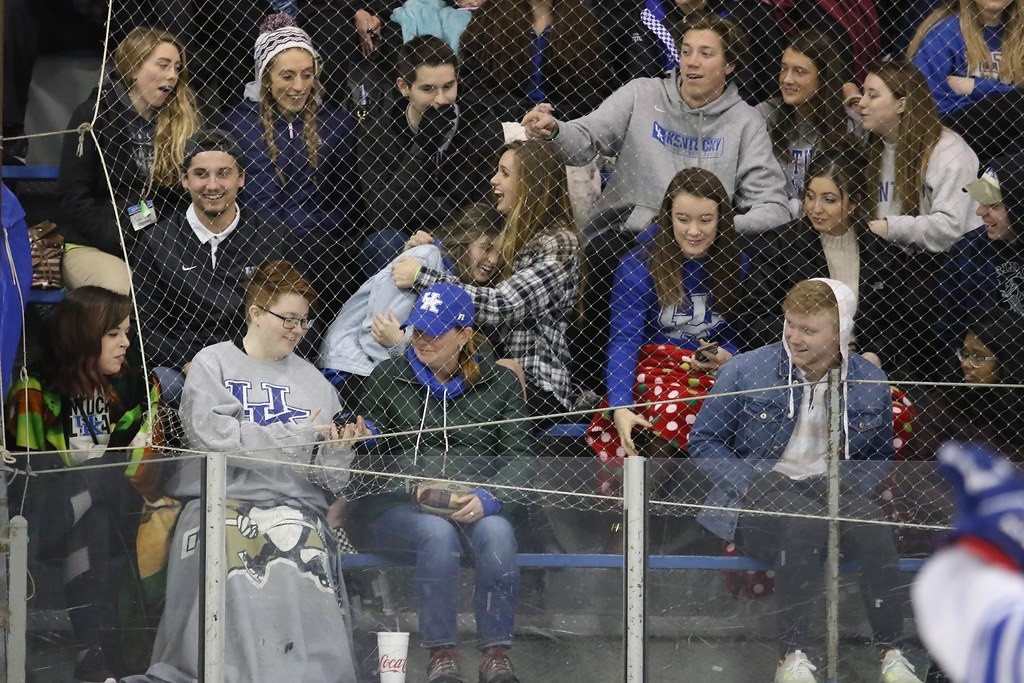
[695,342,718,362]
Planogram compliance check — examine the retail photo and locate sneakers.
[426,650,462,683]
[775,649,817,682]
[479,651,518,683]
[878,649,922,682]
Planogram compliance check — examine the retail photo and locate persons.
[0,0,1024,683]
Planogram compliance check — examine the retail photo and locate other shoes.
[72,648,115,683]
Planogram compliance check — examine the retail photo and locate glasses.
[956,349,997,368]
[254,304,316,329]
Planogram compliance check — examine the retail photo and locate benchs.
[325,423,947,601]
[0,154,65,306]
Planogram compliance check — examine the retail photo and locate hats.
[184,128,240,165]
[247,14,323,107]
[399,283,475,337]
[962,166,1003,206]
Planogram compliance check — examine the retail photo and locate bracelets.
[846,94,862,106]
[545,129,558,140]
[414,266,421,282]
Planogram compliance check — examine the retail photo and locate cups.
[376,631,409,683]
